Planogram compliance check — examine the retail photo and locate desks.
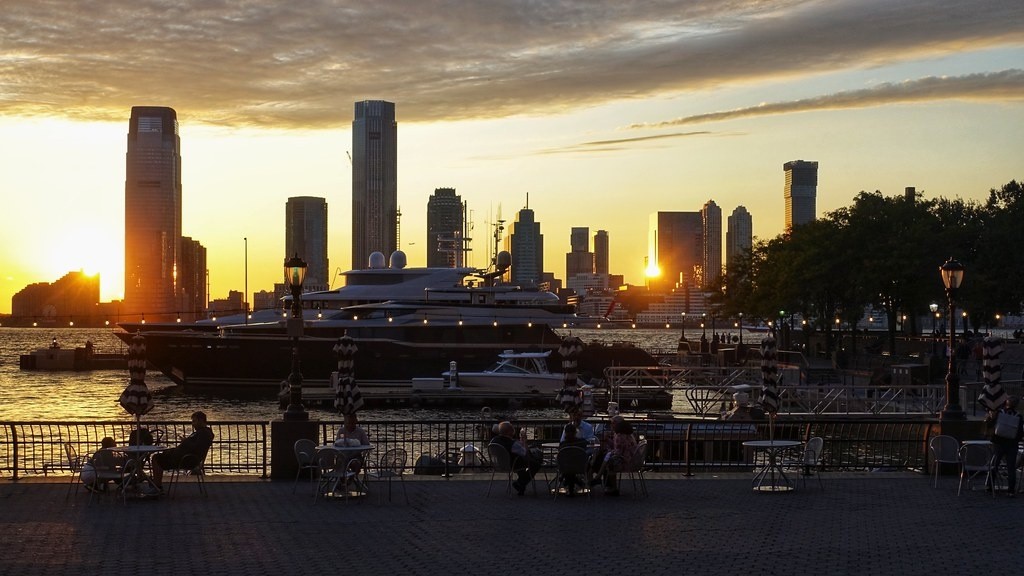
[742,440,805,494]
[106,446,170,497]
[315,445,375,498]
[542,443,600,494]
[963,441,1024,445]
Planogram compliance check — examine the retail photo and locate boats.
[110,246,675,409]
[412,349,565,400]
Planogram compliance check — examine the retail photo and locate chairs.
[293,439,409,507]
[929,435,1024,498]
[487,435,648,500]
[64,443,211,507]
[777,437,824,492]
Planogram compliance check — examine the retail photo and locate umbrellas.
[119,326,155,497]
[759,330,782,492]
[559,335,585,492]
[329,328,364,494]
[978,334,1009,492]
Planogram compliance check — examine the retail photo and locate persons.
[865,375,889,404]
[81,438,117,498]
[559,410,595,449]
[713,332,719,344]
[556,424,586,495]
[720,332,725,343]
[86,341,94,359]
[149,411,216,494]
[488,422,542,496]
[726,331,731,344]
[984,396,1024,497]
[592,417,642,495]
[335,414,370,495]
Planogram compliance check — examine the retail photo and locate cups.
[590,438,595,445]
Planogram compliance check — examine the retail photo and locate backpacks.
[994,409,1020,439]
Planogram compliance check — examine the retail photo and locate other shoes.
[588,478,602,486]
[84,483,99,493]
[144,485,165,496]
[986,482,992,490]
[569,493,574,498]
[605,489,620,497]
[512,482,525,496]
[577,480,585,488]
[1006,489,1014,496]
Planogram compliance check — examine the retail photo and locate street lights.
[737,313,745,346]
[679,311,688,341]
[938,256,968,420]
[279,251,309,421]
[700,313,707,342]
[928,301,940,355]
